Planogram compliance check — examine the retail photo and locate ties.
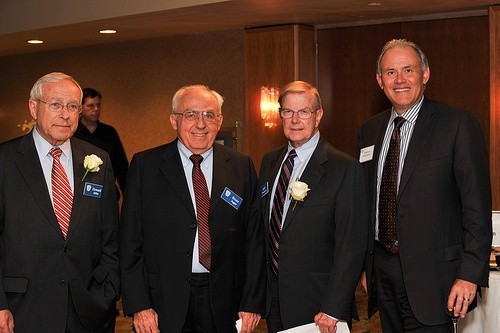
[378,116,407,251]
[48,148,74,240]
[189,154,212,271]
[268,149,297,280]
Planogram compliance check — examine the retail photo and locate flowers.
[289,180,310,211]
[81,154,102,181]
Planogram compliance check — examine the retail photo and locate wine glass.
[443,298,463,333]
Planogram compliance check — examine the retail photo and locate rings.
[463,298,470,301]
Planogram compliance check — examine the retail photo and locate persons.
[358,39,492,333]
[118,85,265,333]
[74,87,129,198]
[260,80,372,332]
[0,72,119,333]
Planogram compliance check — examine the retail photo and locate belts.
[389,245,399,254]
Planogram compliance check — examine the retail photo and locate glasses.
[174,111,220,121]
[38,100,82,115]
[279,108,318,119]
